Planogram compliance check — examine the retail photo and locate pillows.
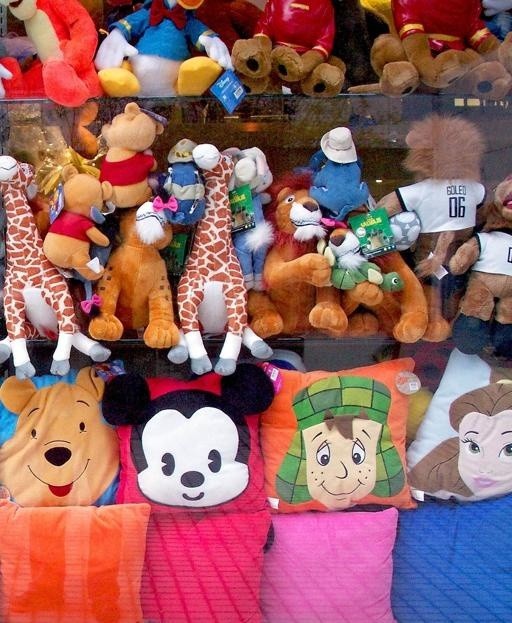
[1,500,151,623]
[140,507,272,623]
[263,507,399,623]
[113,369,263,510]
[262,358,417,513]
[413,345,512,502]
[390,496,512,623]
[0,362,125,509]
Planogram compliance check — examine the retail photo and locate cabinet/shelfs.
[0,1,511,623]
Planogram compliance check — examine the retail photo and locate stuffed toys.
[0,0,512,380]
[1,1,512,382]
